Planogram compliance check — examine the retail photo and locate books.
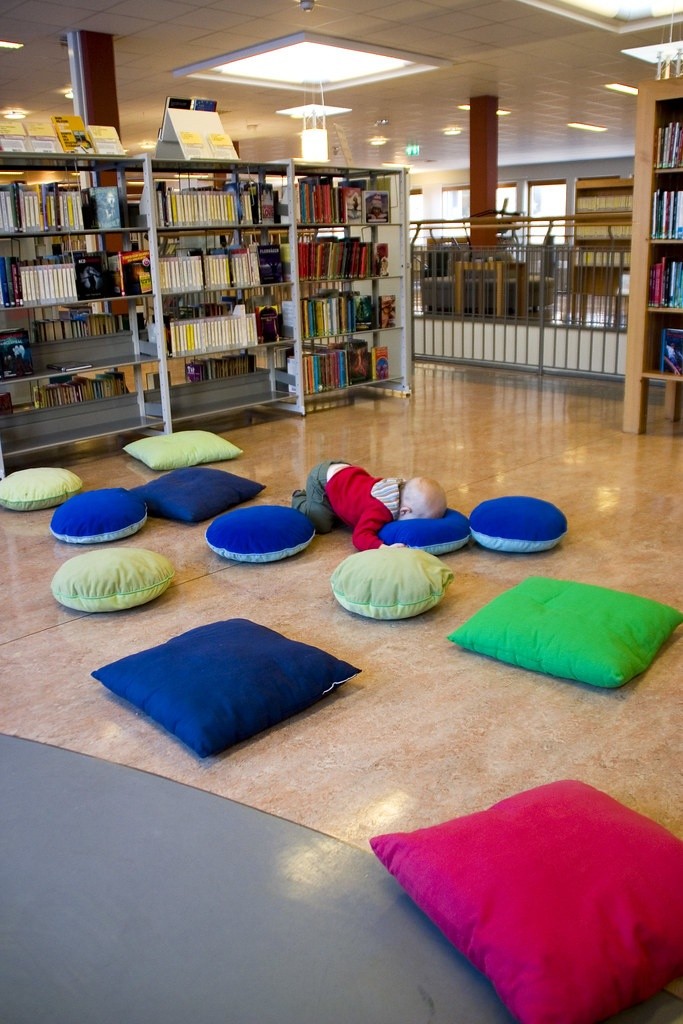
[153,179,282,225]
[648,257,683,309]
[164,287,292,356]
[302,338,389,394]
[50,115,126,155]
[0,305,118,343]
[0,180,86,234]
[186,353,256,383]
[0,368,127,416]
[652,189,683,240]
[576,195,633,213]
[576,226,632,240]
[158,236,285,293]
[655,120,683,168]
[294,175,391,225]
[660,328,683,375]
[1,249,152,308]
[576,250,631,267]
[296,232,389,280]
[299,282,396,339]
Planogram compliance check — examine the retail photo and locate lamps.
[277,0,353,162]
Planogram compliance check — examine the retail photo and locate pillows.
[128,466,266,524]
[122,430,243,471]
[331,547,452,619]
[205,505,314,561]
[469,496,570,552]
[370,780,683,1024]
[0,467,83,513]
[49,488,146,544]
[92,616,362,760]
[375,508,468,555]
[50,548,175,612]
[448,575,683,688]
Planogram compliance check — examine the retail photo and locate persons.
[291,459,446,550]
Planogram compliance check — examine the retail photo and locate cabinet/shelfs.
[622,78,683,436]
[0,151,415,473]
[572,178,634,325]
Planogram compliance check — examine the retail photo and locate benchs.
[421,275,555,313]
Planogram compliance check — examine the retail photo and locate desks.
[456,259,527,317]
